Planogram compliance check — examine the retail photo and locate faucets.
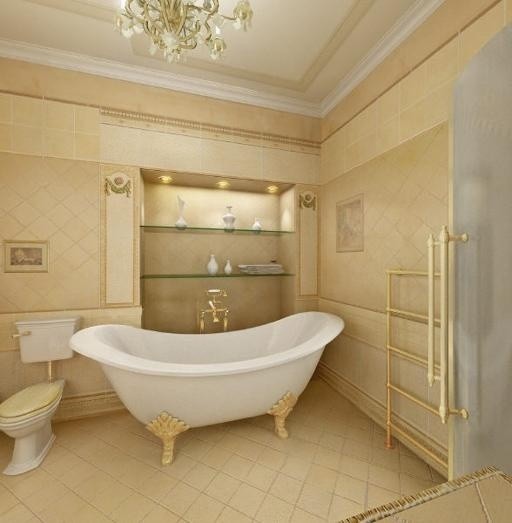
[209,301,220,323]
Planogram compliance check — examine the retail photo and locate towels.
[236,264,285,274]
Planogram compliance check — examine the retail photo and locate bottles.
[253,219,261,230]
[224,259,232,275]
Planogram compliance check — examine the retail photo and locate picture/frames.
[334,192,365,254]
[4,239,51,274]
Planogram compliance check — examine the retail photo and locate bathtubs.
[67,310,345,429]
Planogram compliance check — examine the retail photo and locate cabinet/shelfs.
[140,224,297,279]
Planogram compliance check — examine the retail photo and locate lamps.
[114,0,253,64]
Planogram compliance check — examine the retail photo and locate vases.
[222,206,235,232]
[176,194,187,230]
[252,218,262,235]
[224,260,233,276]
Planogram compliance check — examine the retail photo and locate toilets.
[0,314,82,477]
